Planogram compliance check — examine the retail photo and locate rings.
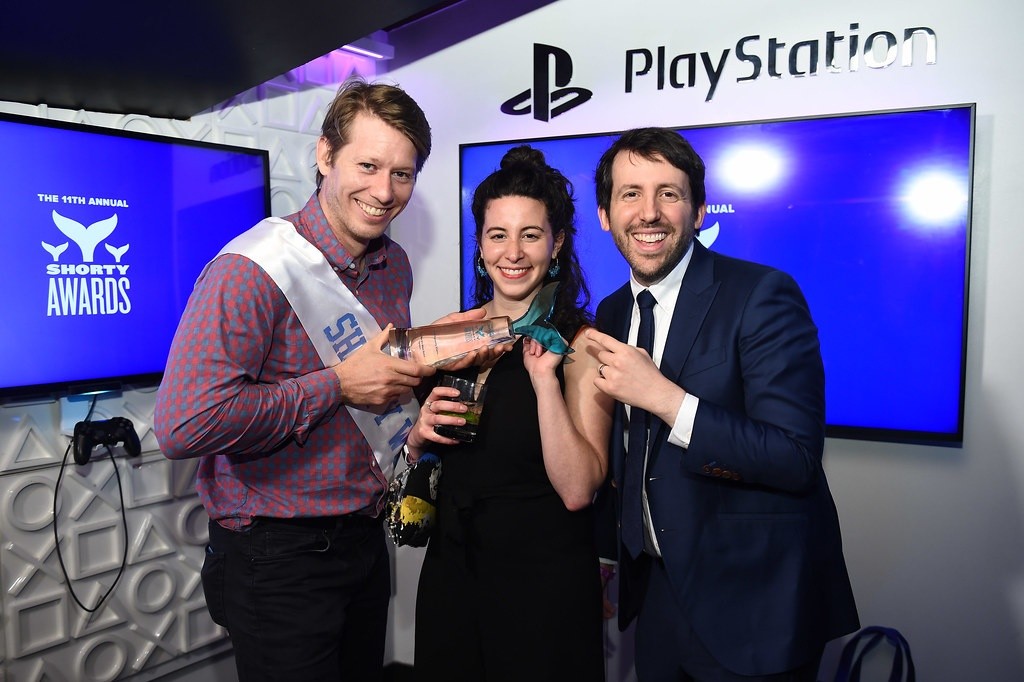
[599,365,608,379]
[427,402,436,413]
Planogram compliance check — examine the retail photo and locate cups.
[434,376,487,443]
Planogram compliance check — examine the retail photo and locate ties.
[620,290,658,560]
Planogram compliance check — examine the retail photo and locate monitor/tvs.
[0,111,273,405]
[459,103,977,445]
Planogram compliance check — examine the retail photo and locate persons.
[400,139,619,682]
[584,127,863,682]
[152,80,512,682]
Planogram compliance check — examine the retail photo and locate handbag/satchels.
[386,452,441,548]
